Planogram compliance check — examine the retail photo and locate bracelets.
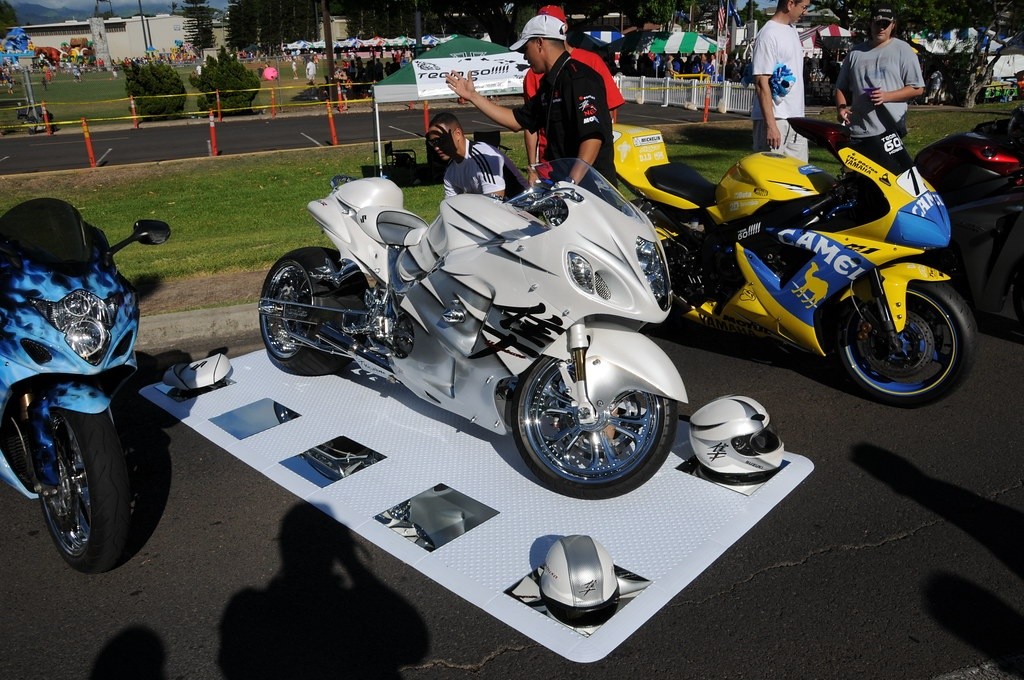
[837,104,847,109]
[565,176,577,184]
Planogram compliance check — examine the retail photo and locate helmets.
[538,536,618,627]
[688,395,784,485]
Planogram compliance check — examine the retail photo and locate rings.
[454,82,455,87]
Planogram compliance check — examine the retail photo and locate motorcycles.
[786,111,1024,335]
[257,156,690,502]
[0,197,173,577]
[612,123,981,410]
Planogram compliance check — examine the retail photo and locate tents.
[617,30,719,54]
[287,34,458,49]
[373,35,533,176]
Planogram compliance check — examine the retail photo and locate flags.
[727,0,742,27]
[717,0,726,35]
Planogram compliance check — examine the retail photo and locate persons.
[750,0,810,166]
[0,51,202,94]
[426,113,530,202]
[802,51,955,105]
[599,49,755,86]
[834,5,925,144]
[446,5,621,194]
[236,47,412,111]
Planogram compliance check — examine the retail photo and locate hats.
[509,15,566,51]
[871,4,896,22]
[538,6,566,23]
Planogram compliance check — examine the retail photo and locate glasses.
[794,2,807,11]
